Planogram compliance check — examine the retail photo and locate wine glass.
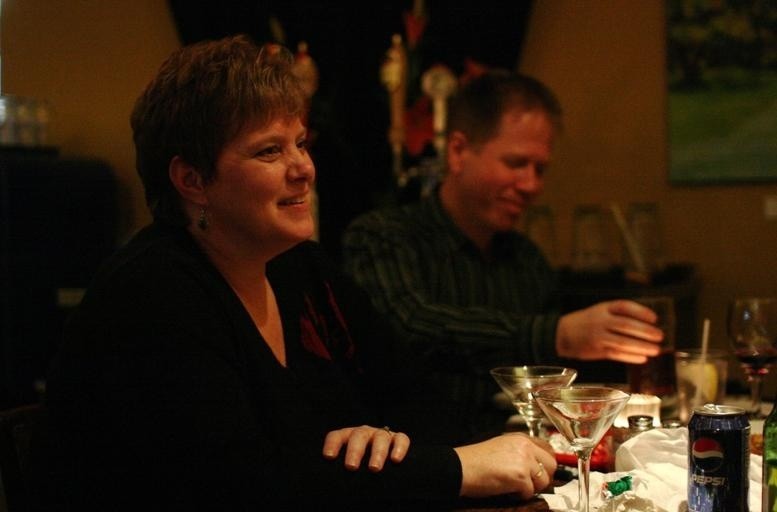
[489,360,631,509]
[727,296,775,418]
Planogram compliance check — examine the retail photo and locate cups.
[631,297,677,359]
[524,202,662,285]
[672,347,733,432]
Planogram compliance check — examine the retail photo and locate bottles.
[609,414,657,474]
[761,385,777,512]
[0,93,50,149]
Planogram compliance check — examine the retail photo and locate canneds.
[687,403,750,512]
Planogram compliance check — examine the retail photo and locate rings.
[380,427,391,437]
[533,463,544,481]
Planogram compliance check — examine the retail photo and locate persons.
[341,70,667,384]
[37,34,557,512]
